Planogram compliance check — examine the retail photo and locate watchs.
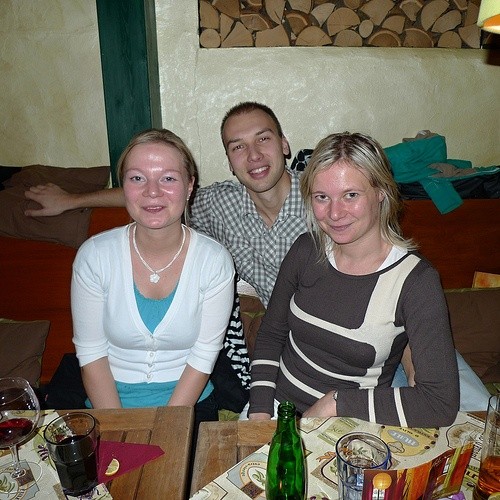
[333,391,337,401]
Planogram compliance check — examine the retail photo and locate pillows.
[0,318,50,389]
[0,165,109,250]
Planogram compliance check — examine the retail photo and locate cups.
[335,431,391,500]
[43,410,98,497]
[473,392,500,500]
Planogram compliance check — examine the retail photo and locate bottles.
[265,401,308,500]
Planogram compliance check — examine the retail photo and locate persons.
[23,102,495,411]
[238,135,460,427]
[72,130,235,409]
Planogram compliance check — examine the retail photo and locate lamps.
[476,0,500,34]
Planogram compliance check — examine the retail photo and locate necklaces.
[133,223,185,282]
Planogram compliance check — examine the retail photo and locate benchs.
[0,164,500,396]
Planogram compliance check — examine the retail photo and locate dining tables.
[0,407,193,500]
[190,411,500,500]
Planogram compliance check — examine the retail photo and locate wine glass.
[0,376,43,494]
